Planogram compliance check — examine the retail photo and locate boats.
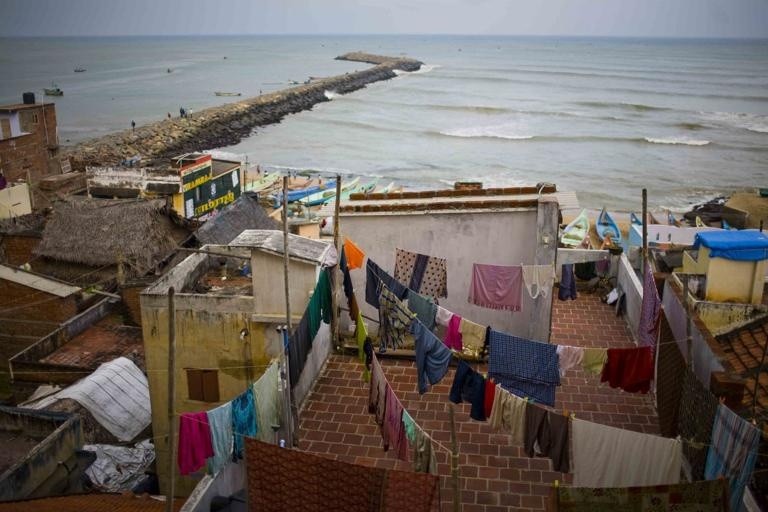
[241,172,395,210]
[44,88,64,96]
[560,206,731,246]
[214,91,242,97]
[759,188,768,196]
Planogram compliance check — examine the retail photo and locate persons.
[179,106,193,119]
[273,169,322,218]
[168,112,171,121]
[243,156,268,184]
[131,120,135,131]
[0,173,7,190]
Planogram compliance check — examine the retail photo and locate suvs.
[684,196,728,221]
